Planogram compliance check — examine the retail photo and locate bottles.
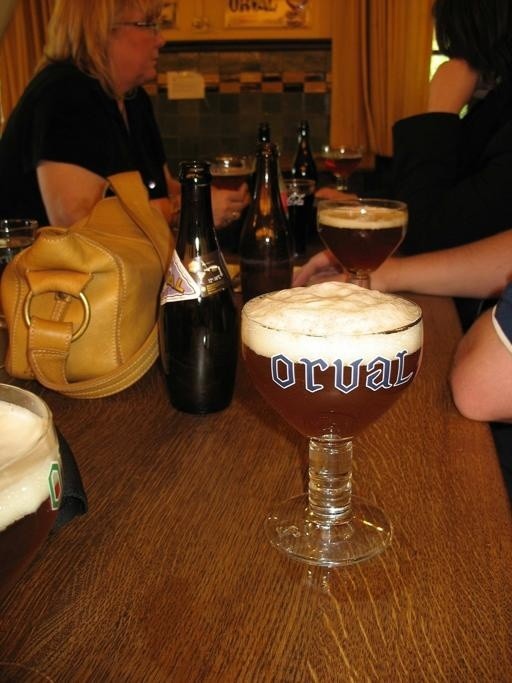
[156,158,241,414]
[241,118,321,321]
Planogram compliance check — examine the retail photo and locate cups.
[0,383,65,590]
[0,219,38,369]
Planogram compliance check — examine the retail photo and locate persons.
[1,0,253,236]
[290,227,512,427]
[311,0,512,254]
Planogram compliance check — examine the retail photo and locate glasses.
[117,15,161,35]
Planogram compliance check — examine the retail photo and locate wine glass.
[320,143,365,193]
[239,278,423,568]
[317,199,408,289]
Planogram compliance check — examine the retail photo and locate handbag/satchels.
[0,170,175,401]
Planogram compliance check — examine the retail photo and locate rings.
[231,212,241,220]
